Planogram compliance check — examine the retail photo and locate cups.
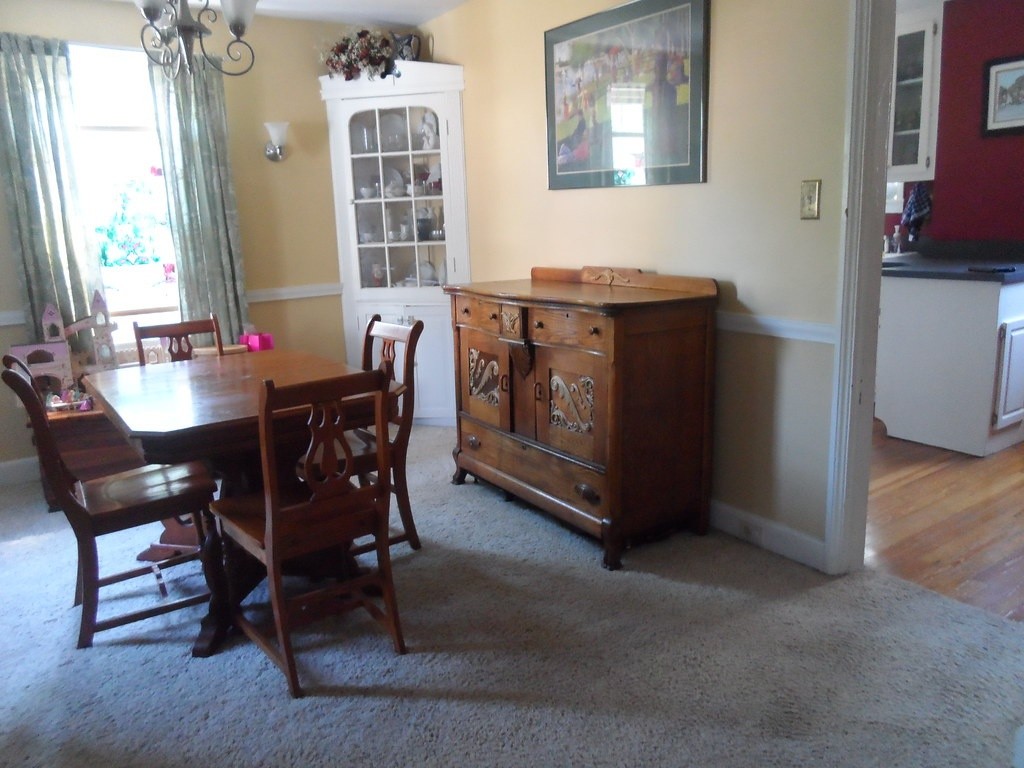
[417,219,431,240]
[400,224,410,240]
[359,127,374,152]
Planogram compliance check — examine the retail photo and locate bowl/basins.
[360,188,376,197]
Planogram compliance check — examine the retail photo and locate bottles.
[371,264,383,287]
[892,225,902,253]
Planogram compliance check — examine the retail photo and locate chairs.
[1,354,238,648]
[295,314,425,561]
[132,313,226,364]
[207,358,408,699]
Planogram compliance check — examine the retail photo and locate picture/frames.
[979,55,1024,138]
[544,1,708,191]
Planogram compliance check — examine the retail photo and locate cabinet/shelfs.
[888,19,937,175]
[446,264,725,572]
[26,400,146,514]
[319,61,472,428]
[991,319,1024,433]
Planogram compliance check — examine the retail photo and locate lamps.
[263,121,289,162]
[136,0,256,86]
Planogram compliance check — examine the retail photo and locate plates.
[374,114,403,145]
[383,167,404,189]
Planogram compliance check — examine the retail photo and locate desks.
[81,346,408,657]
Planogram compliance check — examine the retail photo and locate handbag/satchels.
[240,331,274,351]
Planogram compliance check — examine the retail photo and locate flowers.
[318,29,402,83]
